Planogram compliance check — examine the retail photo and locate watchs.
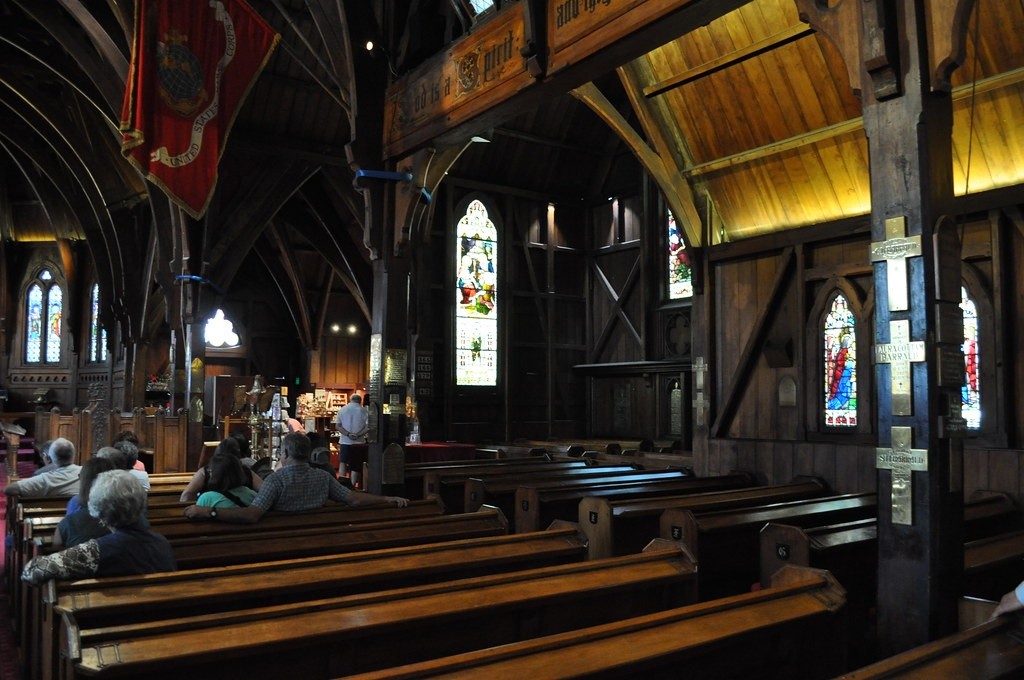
[209,507,217,518]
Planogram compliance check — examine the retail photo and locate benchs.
[9,438,1024,680]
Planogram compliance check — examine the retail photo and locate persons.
[334,395,368,486]
[990,581,1024,619]
[3,428,176,587]
[181,431,411,522]
